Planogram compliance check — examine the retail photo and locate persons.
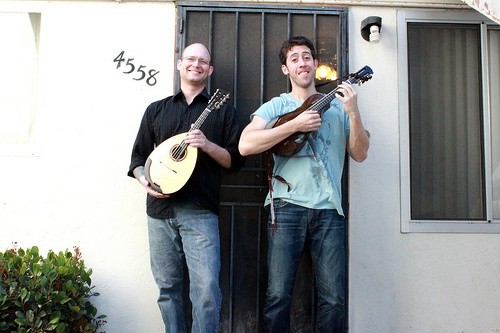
[238,36,370,333]
[127,41,240,332]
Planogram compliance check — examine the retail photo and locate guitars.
[263,64,375,158]
[143,89,231,195]
